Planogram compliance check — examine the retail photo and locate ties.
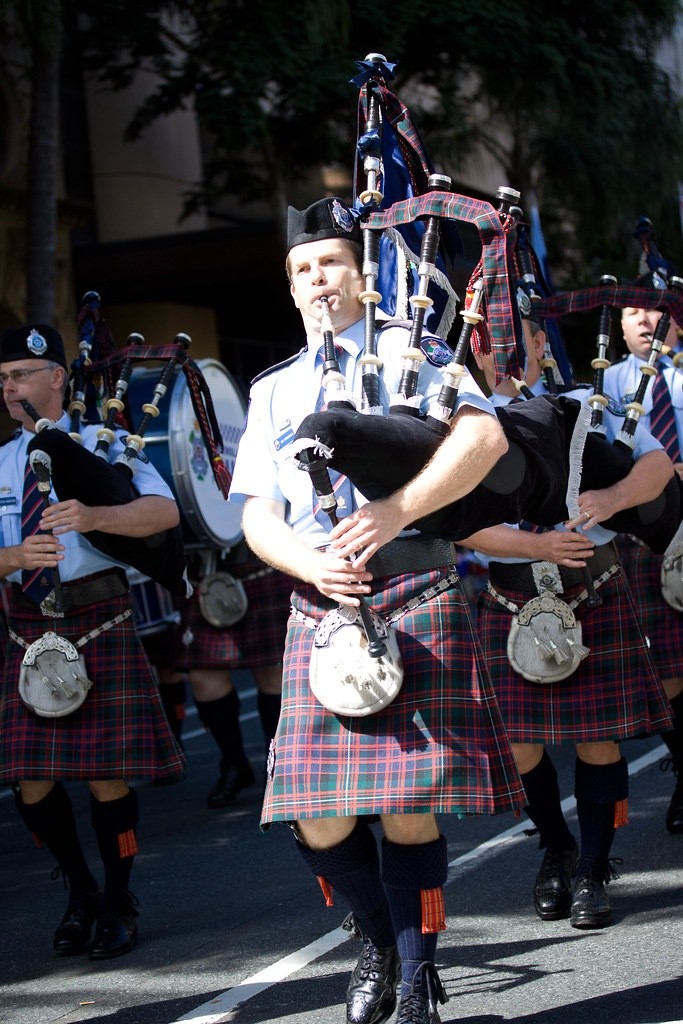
[312,342,352,534]
[650,361,682,465]
[22,455,56,606]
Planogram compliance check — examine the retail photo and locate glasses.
[0,366,60,387]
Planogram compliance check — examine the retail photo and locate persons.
[1,324,181,959]
[228,198,512,1024]
[180,521,292,805]
[601,285,683,837]
[451,282,676,928]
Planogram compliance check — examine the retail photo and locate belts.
[345,535,458,583]
[11,569,130,618]
[489,539,619,597]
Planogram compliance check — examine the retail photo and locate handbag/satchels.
[627,534,683,612]
[486,564,620,684]
[9,608,134,718]
[290,573,458,717]
[186,567,275,628]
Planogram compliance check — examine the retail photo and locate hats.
[482,279,543,328]
[285,197,364,256]
[0,324,68,372]
[634,270,671,313]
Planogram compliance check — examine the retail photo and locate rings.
[584,512,589,519]
[355,537,363,550]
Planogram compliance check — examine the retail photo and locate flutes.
[18,292,193,612]
[293,54,683,657]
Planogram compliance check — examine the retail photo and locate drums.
[122,358,248,558]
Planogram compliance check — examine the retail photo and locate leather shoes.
[570,877,614,926]
[346,938,402,1024]
[396,993,442,1024]
[55,886,104,955]
[207,761,257,809]
[666,792,683,833]
[534,834,579,919]
[90,912,141,960]
